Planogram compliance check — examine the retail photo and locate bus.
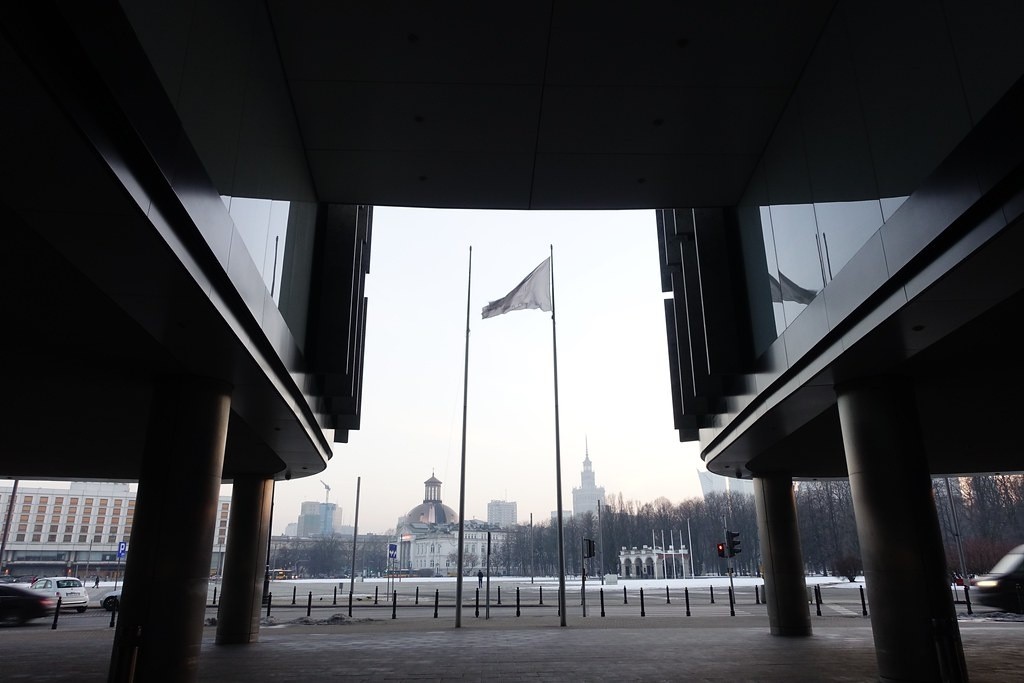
[268,569,293,579]
[383,568,419,578]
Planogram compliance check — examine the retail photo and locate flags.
[778,269,817,305]
[768,272,783,303]
[481,260,554,319]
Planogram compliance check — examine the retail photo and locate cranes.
[320,479,331,504]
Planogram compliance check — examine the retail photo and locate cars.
[971,543,1024,614]
[0,584,55,627]
[100,589,122,611]
[30,576,89,613]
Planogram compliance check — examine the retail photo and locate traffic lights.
[727,531,741,557]
[583,539,595,558]
[717,544,725,558]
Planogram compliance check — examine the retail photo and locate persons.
[31,575,38,585]
[477,570,484,589]
[91,576,99,588]
